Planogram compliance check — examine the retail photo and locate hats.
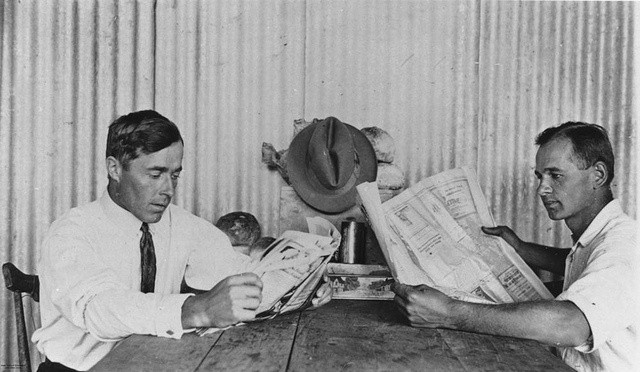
[287,117,377,215]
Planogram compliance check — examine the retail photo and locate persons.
[30,109,334,371]
[388,120,639,370]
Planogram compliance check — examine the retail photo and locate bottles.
[339,221,366,264]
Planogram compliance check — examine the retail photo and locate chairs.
[3,262,39,372]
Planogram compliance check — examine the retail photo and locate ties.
[140,222,157,293]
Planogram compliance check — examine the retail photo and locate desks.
[91,300,578,372]
